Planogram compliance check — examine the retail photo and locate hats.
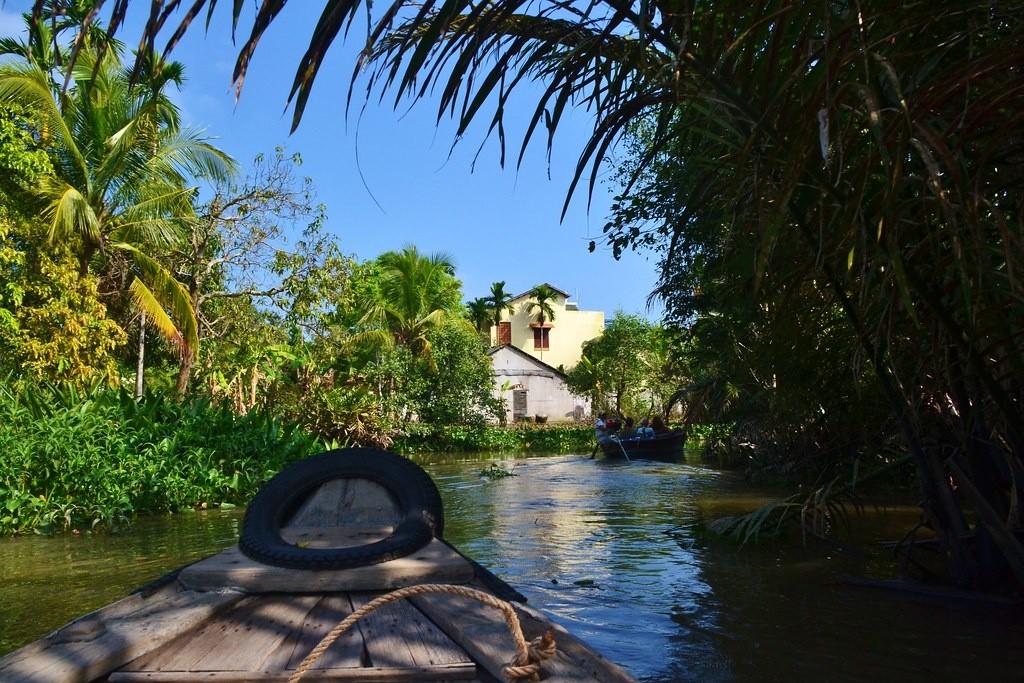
[641,418,648,424]
[599,411,607,416]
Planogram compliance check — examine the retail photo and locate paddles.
[590,443,599,459]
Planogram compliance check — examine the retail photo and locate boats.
[596,422,690,458]
[0,447,642,683]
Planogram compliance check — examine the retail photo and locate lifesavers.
[236,446,448,570]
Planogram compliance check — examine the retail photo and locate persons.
[652,416,672,434]
[619,417,637,438]
[636,418,655,437]
[595,410,617,444]
